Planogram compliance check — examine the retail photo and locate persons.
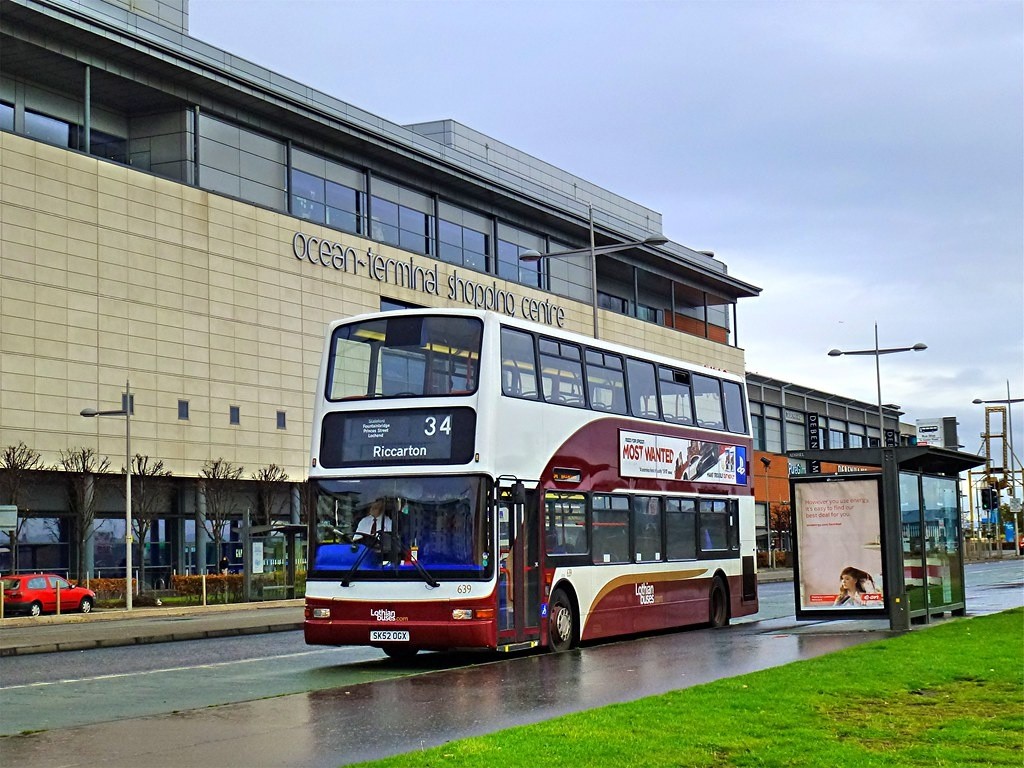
[856,570,883,606]
[353,497,392,542]
[219,556,230,575]
[833,567,860,606]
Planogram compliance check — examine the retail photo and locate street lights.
[78,379,135,608]
[827,320,929,447]
[518,203,668,339]
[973,379,1024,556]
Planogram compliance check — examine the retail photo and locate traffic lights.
[991,490,998,510]
[980,489,992,510]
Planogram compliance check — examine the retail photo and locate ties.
[371,519,378,535]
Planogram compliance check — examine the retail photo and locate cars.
[1,573,96,617]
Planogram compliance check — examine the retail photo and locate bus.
[296,304,761,661]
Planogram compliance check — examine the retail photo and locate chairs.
[449,384,732,557]
